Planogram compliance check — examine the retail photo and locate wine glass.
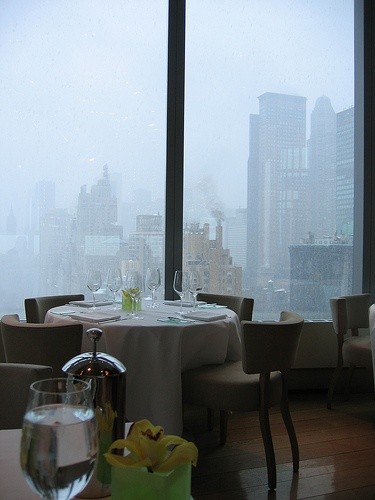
[145,268,161,309]
[189,272,202,313]
[128,272,145,320]
[87,270,102,310]
[20,378,99,500]
[107,269,122,309]
[173,271,188,315]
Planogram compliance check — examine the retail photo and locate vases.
[111,453,191,500]
[121,300,142,311]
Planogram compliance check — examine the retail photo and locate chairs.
[0,314,83,366]
[0,363,53,429]
[25,294,84,324]
[327,293,373,410]
[197,293,254,441]
[181,311,304,489]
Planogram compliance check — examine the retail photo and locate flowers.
[121,288,141,299]
[102,420,199,474]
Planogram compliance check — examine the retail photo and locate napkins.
[72,312,121,322]
[182,311,227,320]
[164,300,206,307]
[68,301,112,306]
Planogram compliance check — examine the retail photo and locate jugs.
[61,328,126,498]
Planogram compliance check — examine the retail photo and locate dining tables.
[45,301,242,439]
